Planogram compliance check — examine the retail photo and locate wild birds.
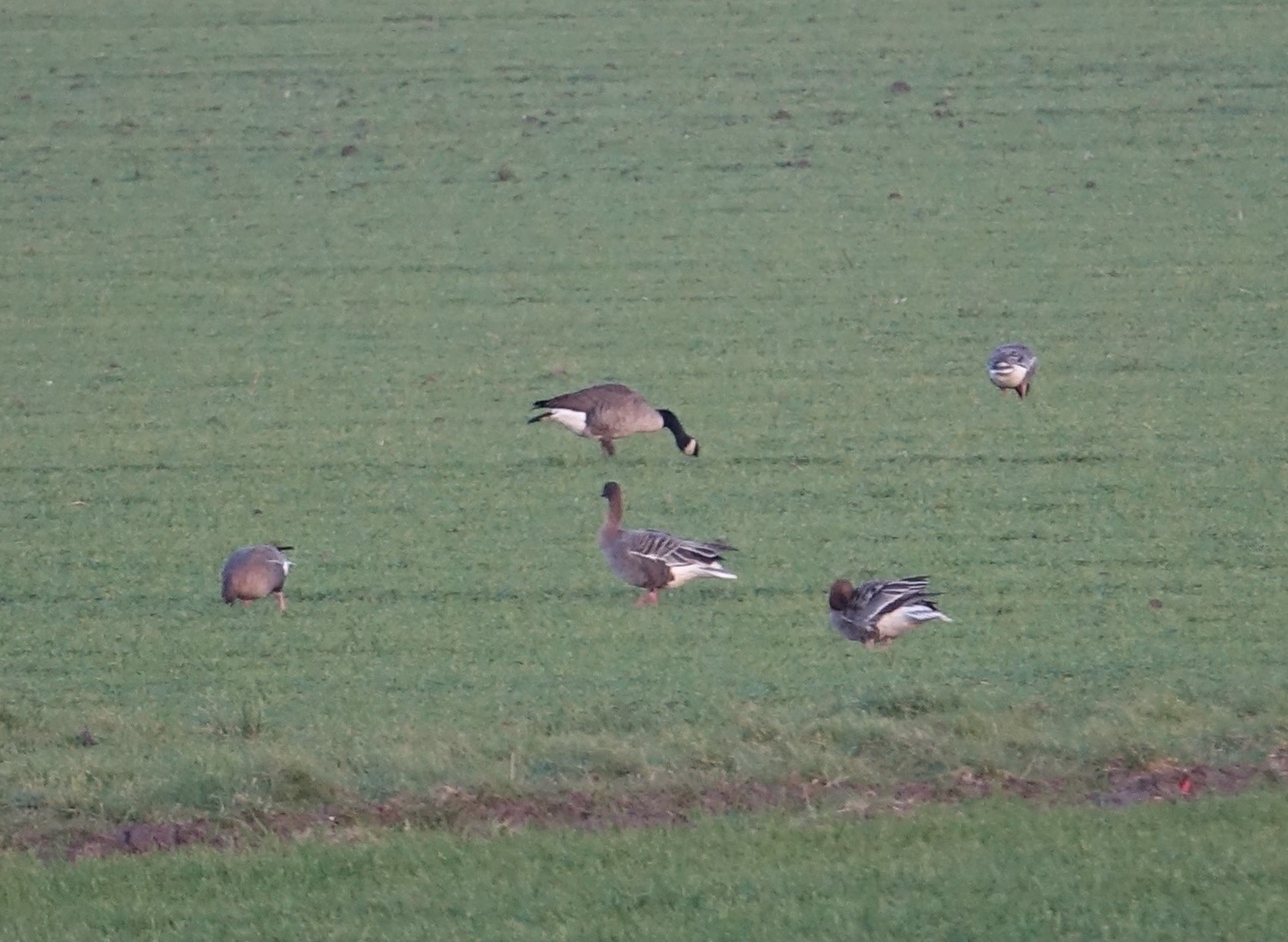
[987,343,1037,397]
[598,481,739,601]
[527,384,699,459]
[824,574,955,642]
[222,541,296,610]
